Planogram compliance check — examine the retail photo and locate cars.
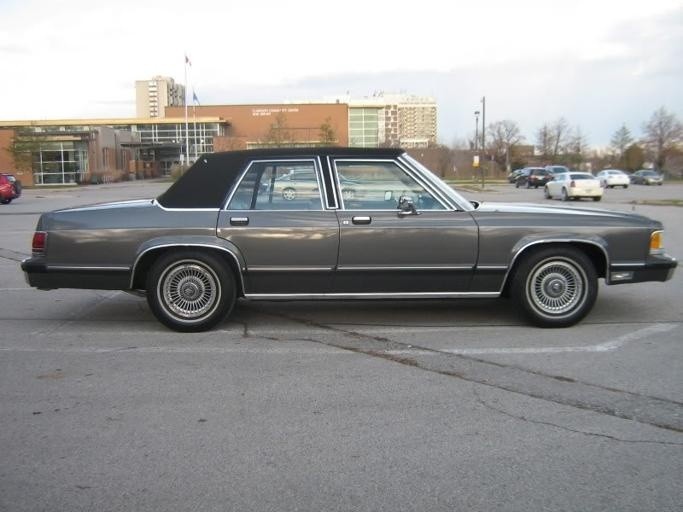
[540,170,605,204]
[505,158,667,190]
[262,165,374,202]
[16,141,680,334]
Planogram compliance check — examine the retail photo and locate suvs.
[0,171,22,205]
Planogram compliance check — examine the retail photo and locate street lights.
[474,110,481,182]
[479,94,486,189]
